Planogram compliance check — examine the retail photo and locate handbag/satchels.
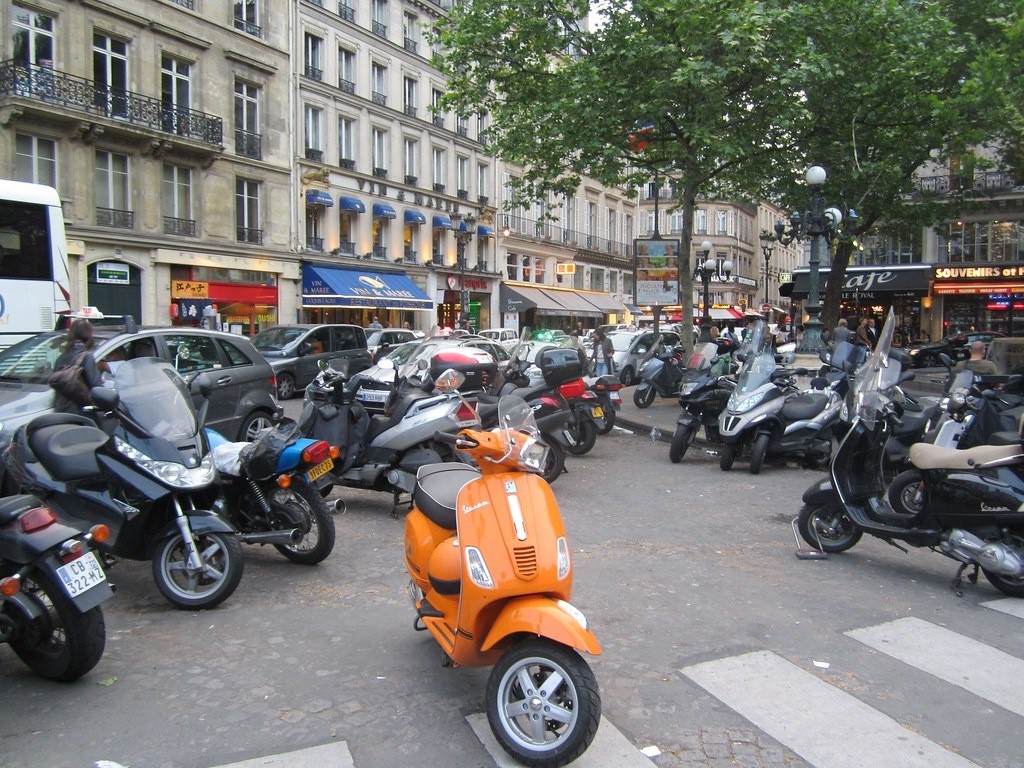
[49,352,92,406]
[732,338,740,350]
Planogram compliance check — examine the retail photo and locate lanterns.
[170,303,178,320]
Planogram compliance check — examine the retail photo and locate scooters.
[0,346,625,768]
[633,305,1024,599]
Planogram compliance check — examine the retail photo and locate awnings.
[500,284,643,318]
[934,283,1024,295]
[302,264,433,313]
[669,307,789,319]
[791,268,931,297]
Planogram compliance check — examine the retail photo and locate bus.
[0,176,71,372]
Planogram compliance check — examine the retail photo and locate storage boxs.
[429,347,498,391]
[540,348,590,389]
[888,350,913,372]
[712,337,733,354]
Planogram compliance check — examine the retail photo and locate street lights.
[758,228,774,305]
[774,165,858,355]
[692,240,733,344]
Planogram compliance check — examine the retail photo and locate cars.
[250,323,375,402]
[905,330,1009,368]
[453,321,701,387]
[343,333,512,423]
[0,305,279,503]
[363,327,418,366]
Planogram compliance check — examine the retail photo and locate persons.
[589,328,614,377]
[462,320,474,335]
[51,320,110,435]
[713,317,878,354]
[956,341,1000,391]
[573,322,585,336]
[304,334,322,354]
[369,316,410,329]
[98,346,130,388]
